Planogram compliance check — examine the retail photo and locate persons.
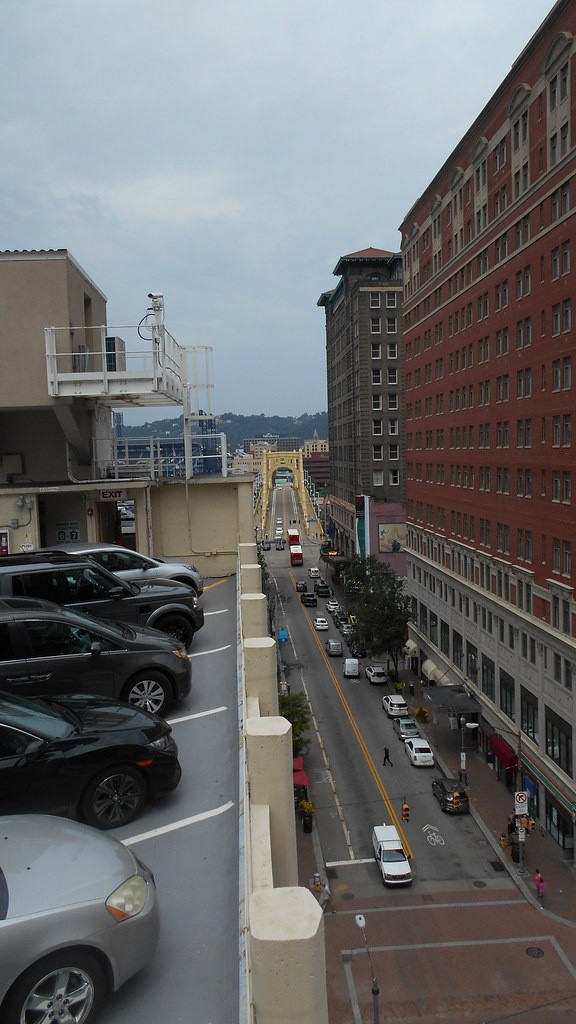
[382,745,393,767]
[534,868,545,910]
[311,532,324,540]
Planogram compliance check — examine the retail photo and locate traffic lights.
[401,803,410,823]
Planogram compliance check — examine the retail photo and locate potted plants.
[394,681,405,694]
[387,669,397,681]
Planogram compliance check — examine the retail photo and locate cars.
[295,581,307,592]
[430,777,469,814]
[404,737,434,767]
[0,814,161,1024]
[326,601,355,637]
[392,717,420,740]
[365,665,387,684]
[23,542,203,597]
[0,595,191,716]
[300,593,318,607]
[308,568,320,579]
[381,695,408,718]
[0,689,183,831]
[314,579,330,598]
[274,517,284,550]
[325,639,343,657]
[262,541,271,551]
[313,617,329,631]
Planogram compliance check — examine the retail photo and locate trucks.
[342,658,361,677]
[372,825,413,889]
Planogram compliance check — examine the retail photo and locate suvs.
[0,550,205,651]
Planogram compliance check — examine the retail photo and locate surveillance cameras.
[148,293,163,298]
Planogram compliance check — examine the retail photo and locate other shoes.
[540,905,544,910]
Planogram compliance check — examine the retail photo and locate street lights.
[354,914,380,1024]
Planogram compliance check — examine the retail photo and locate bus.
[289,544,303,566]
[286,528,300,544]
